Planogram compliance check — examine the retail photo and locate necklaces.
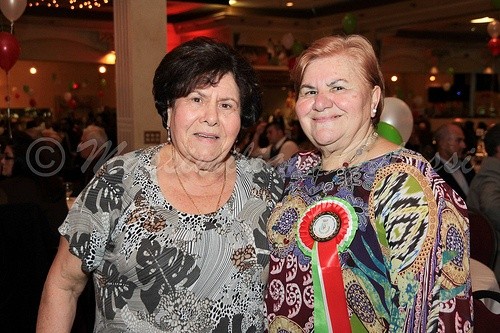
[171,151,226,220]
[313,133,377,182]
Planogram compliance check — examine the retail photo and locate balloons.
[488,21,500,58]
[100,78,106,87]
[62,81,79,110]
[5,85,31,101]
[97,89,104,97]
[374,96,413,147]
[29,99,36,108]
[0,0,27,23]
[0,32,20,72]
[342,13,356,33]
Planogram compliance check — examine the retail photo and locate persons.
[468,123,500,282]
[0,131,41,203]
[0,105,116,145]
[428,124,476,200]
[249,122,299,168]
[41,124,113,198]
[264,34,473,333]
[35,38,282,333]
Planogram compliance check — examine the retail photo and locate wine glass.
[65,183,73,201]
